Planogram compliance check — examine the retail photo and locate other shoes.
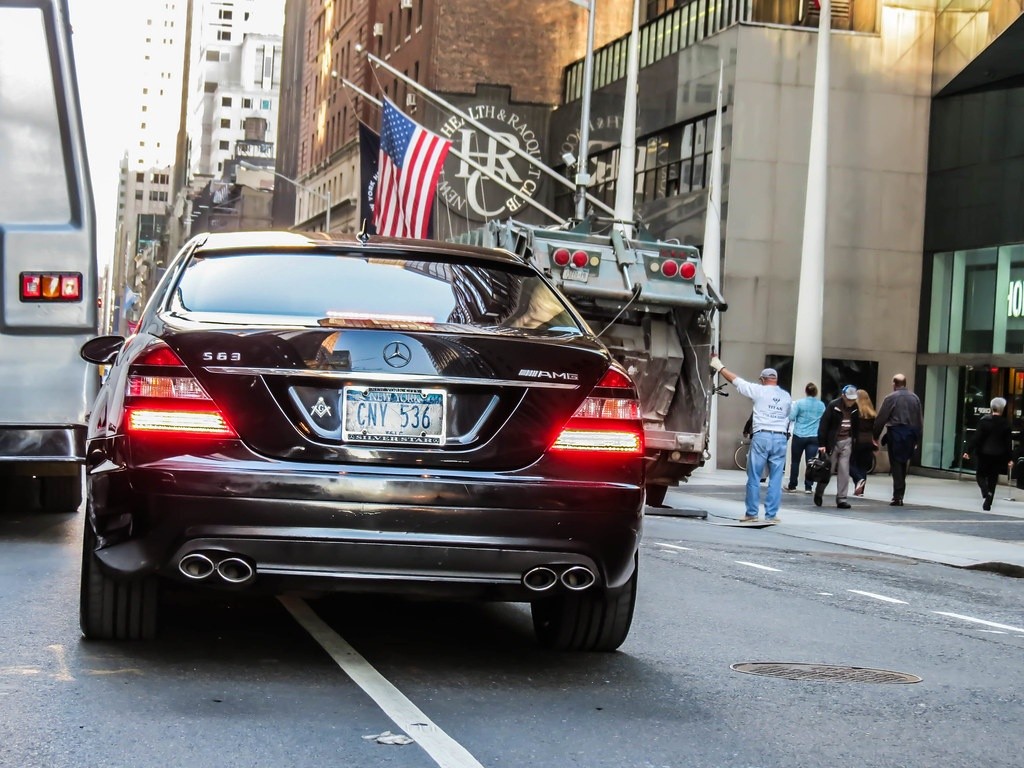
[760,478,767,483]
[805,490,811,493]
[854,479,866,496]
[837,502,851,509]
[783,484,796,492]
[739,516,758,522]
[983,492,992,510]
[889,500,903,506]
[765,517,781,523]
[814,492,823,506]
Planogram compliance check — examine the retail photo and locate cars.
[75,227,653,657]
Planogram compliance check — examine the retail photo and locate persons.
[782,382,825,494]
[849,389,878,497]
[963,397,1014,512]
[813,385,859,509]
[872,373,923,507]
[710,356,791,522]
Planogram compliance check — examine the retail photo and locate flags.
[124,286,136,311]
[370,94,455,240]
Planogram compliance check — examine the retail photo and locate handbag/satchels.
[807,450,831,484]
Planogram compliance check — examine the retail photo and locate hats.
[842,385,857,400]
[759,369,777,379]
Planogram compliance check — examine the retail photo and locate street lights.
[240,159,331,232]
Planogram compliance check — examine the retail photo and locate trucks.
[439,215,731,508]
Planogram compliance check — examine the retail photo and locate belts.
[756,430,786,435]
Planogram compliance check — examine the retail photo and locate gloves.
[710,356,725,373]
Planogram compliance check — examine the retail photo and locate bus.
[2,0,104,520]
